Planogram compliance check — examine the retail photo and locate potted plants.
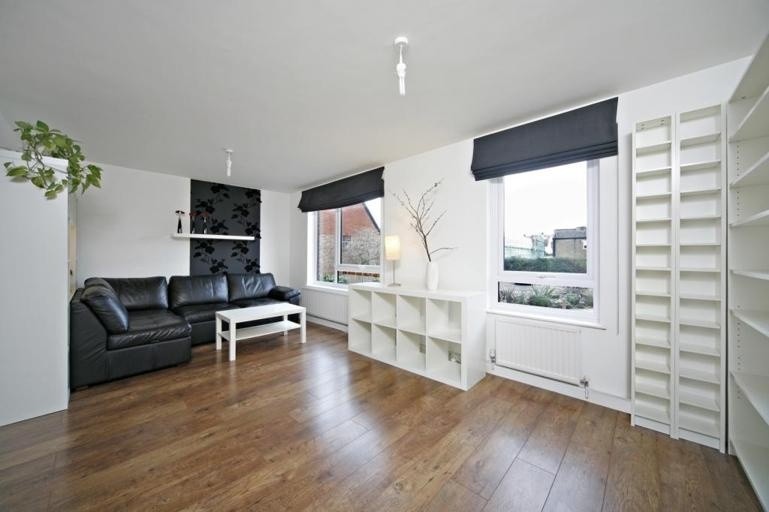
[389,177,461,290]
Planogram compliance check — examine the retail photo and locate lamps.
[394,36,408,96]
[225,149,233,176]
[384,234,401,286]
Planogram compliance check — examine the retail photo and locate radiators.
[489,317,589,387]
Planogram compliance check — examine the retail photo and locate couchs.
[70,288,192,391]
[170,273,301,344]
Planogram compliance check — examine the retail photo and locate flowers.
[200,211,209,217]
[189,210,198,216]
[175,210,185,215]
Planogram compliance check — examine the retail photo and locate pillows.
[84,276,169,309]
[226,273,276,302]
[79,285,128,334]
[169,274,229,310]
[85,278,119,297]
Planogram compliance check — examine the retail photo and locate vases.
[203,217,208,234]
[191,215,196,234]
[177,210,182,234]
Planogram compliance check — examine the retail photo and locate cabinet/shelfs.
[727,32,769,512]
[631,100,727,454]
[348,282,487,391]
